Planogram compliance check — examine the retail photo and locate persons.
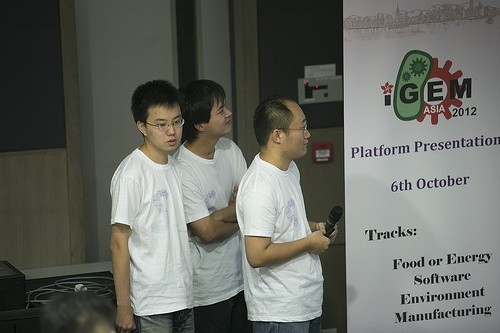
[39,290,118,333]
[173,80,253,333]
[235,95,339,333]
[110,80,194,333]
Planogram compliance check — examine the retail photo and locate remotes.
[324,227,335,237]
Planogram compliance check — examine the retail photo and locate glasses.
[271,124,308,133]
[137,120,184,131]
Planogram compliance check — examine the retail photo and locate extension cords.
[74,283,88,292]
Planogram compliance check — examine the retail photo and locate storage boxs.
[0,260,26,312]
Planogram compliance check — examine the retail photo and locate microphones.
[324,206,343,232]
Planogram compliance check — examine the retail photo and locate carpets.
[26,271,117,308]
